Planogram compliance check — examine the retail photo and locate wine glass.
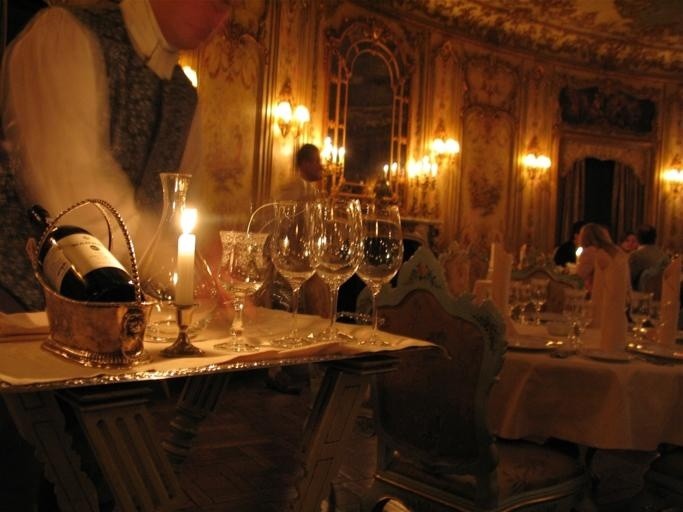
[212,192,402,353]
[629,291,654,338]
[473,277,593,344]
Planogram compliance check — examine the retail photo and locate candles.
[172,201,200,306]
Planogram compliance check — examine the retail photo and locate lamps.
[376,161,405,202]
[408,114,461,217]
[269,73,311,138]
[320,136,348,191]
[661,152,682,196]
[519,135,554,188]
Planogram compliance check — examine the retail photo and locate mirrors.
[327,15,411,202]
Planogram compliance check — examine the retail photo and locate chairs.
[353,222,682,511]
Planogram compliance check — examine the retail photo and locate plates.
[582,342,683,363]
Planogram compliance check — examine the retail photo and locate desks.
[0,300,441,511]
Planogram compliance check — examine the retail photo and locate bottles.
[127,172,214,347]
[657,246,681,344]
[29,204,143,305]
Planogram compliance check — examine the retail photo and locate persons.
[289,144,325,198]
[551,220,666,305]
[0,0,258,329]
